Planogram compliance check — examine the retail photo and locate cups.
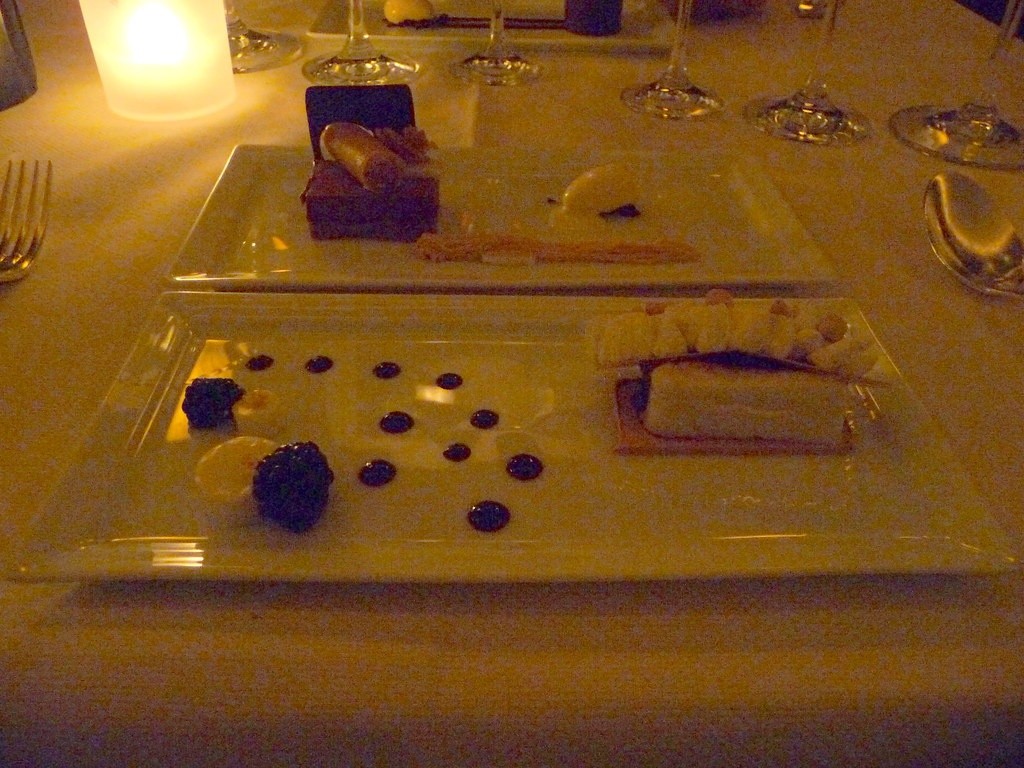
[0,0,37,112]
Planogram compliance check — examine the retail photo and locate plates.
[0,289,1024,583]
[306,0,682,52]
[161,144,836,284]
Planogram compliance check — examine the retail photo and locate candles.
[78,0,235,123]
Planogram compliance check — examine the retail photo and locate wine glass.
[743,0,872,147]
[446,0,548,87]
[620,0,726,118]
[223,0,302,73]
[302,0,421,87]
[889,0,1024,170]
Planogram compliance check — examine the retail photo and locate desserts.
[562,0,626,37]
[293,83,440,242]
[385,0,448,29]
[589,290,881,449]
[563,162,646,219]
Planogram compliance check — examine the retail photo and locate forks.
[0,161,52,283]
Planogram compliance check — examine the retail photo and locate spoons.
[923,171,1024,302]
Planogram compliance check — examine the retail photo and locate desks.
[0,0,1023,768]
